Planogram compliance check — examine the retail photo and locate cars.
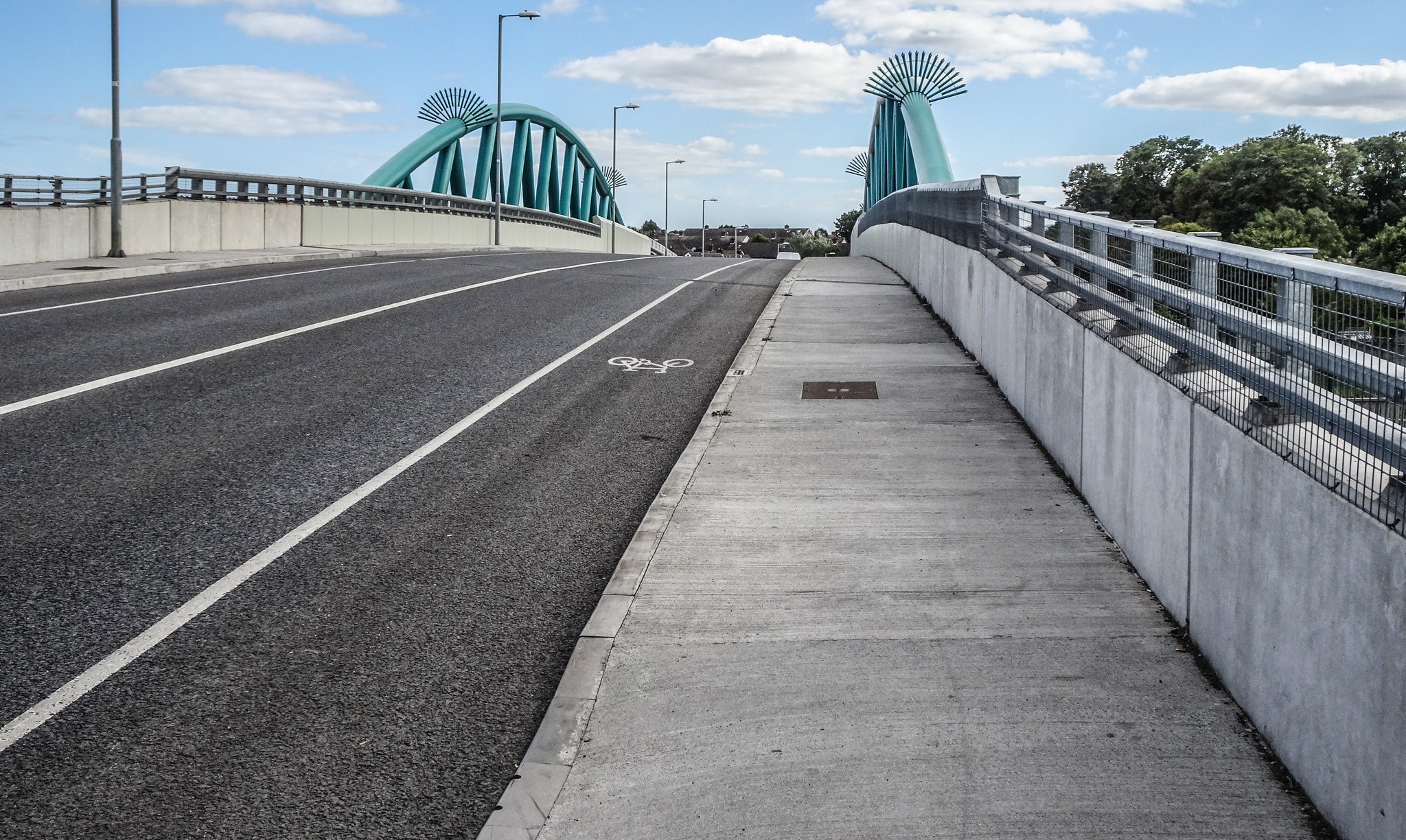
[776,252,802,260]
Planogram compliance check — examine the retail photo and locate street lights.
[777,242,789,253]
[825,252,836,257]
[735,226,744,258]
[492,10,541,246]
[665,159,686,257]
[611,103,641,253]
[702,198,719,258]
